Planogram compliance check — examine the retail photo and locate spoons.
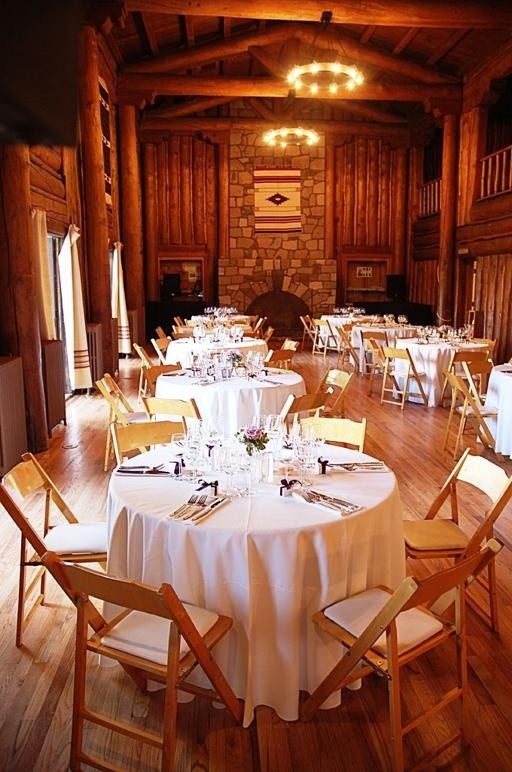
[119,462,166,470]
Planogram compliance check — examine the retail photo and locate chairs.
[299,534,503,771]
[0,452,107,647]
[41,551,243,772]
[300,313,511,463]
[94,314,367,473]
[403,446,512,634]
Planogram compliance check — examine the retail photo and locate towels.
[113,459,173,476]
[168,493,230,526]
[327,457,390,473]
[290,483,363,517]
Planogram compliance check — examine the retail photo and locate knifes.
[184,496,228,526]
[117,469,170,477]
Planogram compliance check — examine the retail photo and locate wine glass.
[332,306,366,318]
[169,414,325,496]
[193,325,247,345]
[416,326,472,345]
[369,313,409,328]
[187,350,269,382]
[204,306,238,316]
[190,316,247,326]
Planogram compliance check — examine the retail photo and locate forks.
[168,494,209,518]
[302,489,359,515]
[330,459,385,473]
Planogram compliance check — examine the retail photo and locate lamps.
[261,97,320,149]
[285,11,364,96]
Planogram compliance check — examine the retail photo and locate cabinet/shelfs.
[85,324,103,393]
[40,340,67,438]
[1,356,29,471]
[128,315,138,356]
[100,317,120,379]
